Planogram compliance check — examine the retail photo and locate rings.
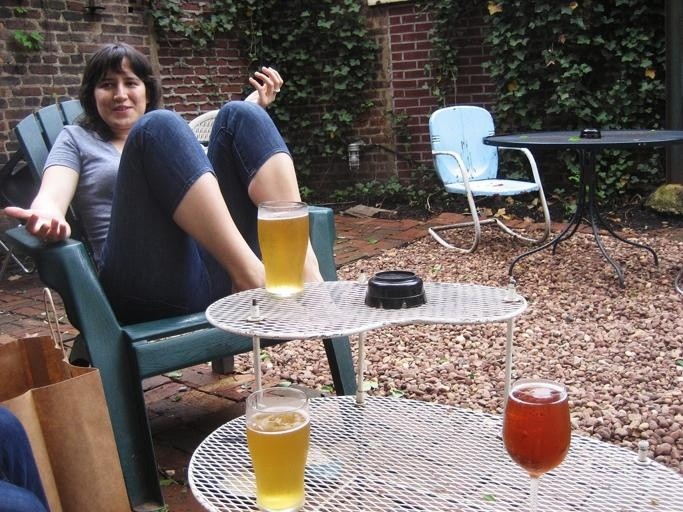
[273,88,281,92]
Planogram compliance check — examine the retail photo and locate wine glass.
[501,379,571,512]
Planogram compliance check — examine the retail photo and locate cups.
[256,200,309,296]
[245,386,310,512]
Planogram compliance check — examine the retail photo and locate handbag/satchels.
[0,287,132,511]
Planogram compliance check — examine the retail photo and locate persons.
[1,43,354,335]
[0,408,48,512]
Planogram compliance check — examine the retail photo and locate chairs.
[185,108,222,157]
[2,94,365,512]
[426,101,552,255]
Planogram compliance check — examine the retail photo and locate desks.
[188,276,683,511]
[482,130,683,288]
